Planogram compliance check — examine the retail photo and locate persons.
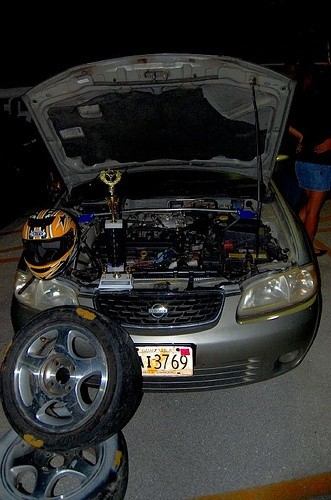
[272,59,331,257]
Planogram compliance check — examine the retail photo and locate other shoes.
[313,248,328,257]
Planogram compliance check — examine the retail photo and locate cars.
[10,53,324,395]
